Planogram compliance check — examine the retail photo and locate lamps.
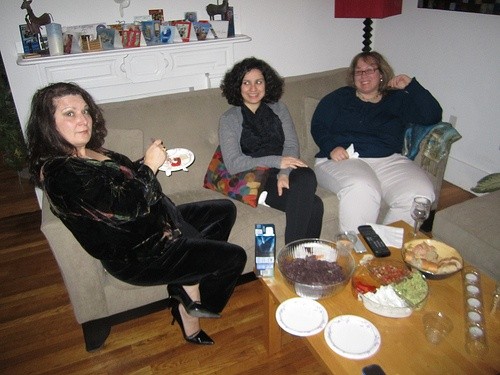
[334,0,403,53]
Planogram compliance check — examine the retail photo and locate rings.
[163,146,167,151]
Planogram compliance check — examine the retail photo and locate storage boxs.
[255,262,275,276]
[255,223,276,263]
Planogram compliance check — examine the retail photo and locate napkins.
[364,221,405,249]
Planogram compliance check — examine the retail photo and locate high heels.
[170,304,215,344]
[166,282,222,320]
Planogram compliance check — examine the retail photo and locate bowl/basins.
[275,238,355,299]
[402,239,463,281]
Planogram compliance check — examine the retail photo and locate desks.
[15,33,253,105]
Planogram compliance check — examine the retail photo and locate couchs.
[40,67,463,354]
[431,187,500,285]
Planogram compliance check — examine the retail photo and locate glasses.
[352,67,379,76]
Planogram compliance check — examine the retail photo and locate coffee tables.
[253,219,500,375]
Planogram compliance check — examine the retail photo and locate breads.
[438,257,463,275]
[418,258,438,273]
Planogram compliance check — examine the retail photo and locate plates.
[352,257,430,318]
[324,315,381,360]
[275,297,329,336]
[158,148,194,176]
[294,242,337,262]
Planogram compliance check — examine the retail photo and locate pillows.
[95,129,144,162]
[304,96,321,161]
[202,143,275,209]
[470,172,500,193]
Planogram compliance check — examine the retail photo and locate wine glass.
[408,197,431,239]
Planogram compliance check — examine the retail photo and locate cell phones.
[362,364,385,375]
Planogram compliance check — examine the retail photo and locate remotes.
[344,230,367,254]
[358,225,390,257]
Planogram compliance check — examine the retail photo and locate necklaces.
[360,92,379,100]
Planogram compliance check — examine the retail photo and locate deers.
[206,0,228,20]
[21,0,54,34]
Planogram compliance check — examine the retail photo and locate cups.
[46,23,64,56]
[422,311,453,344]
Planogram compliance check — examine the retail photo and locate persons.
[312,52,442,234]
[217,57,323,249]
[24,82,247,345]
